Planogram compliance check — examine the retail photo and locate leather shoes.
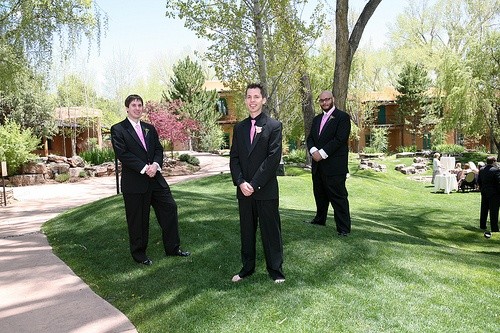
[139,256,153,266]
[172,249,190,257]
[303,220,326,226]
[338,232,351,237]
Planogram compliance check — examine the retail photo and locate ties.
[319,107,334,133]
[135,125,147,151]
[250,119,256,144]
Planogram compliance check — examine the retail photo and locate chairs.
[459,172,475,192]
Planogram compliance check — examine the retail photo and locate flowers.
[327,115,335,122]
[144,129,150,137]
[255,125,262,137]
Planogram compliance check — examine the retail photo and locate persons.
[431,153,442,184]
[304,90,352,237]
[229,83,286,283]
[111,94,191,265]
[478,156,500,232]
[449,161,485,193]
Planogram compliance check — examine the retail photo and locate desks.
[435,174,459,193]
